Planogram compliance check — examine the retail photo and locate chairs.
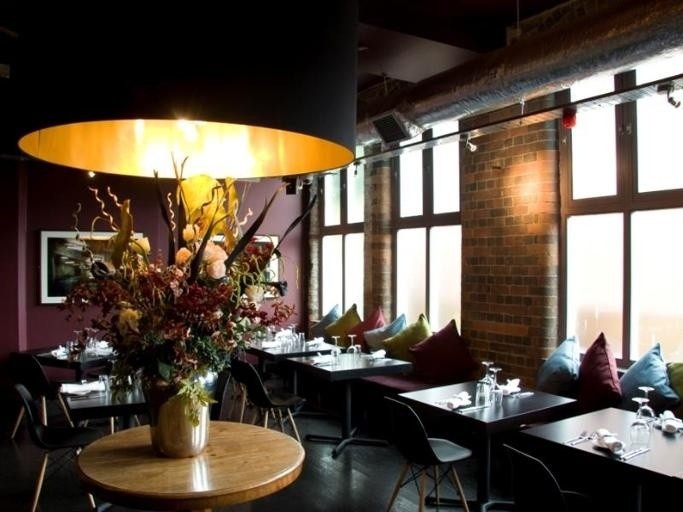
[12,350,100,512]
[211,361,306,444]
[385,397,577,512]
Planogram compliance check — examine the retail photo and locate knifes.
[620,444,650,461]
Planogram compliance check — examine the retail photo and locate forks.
[571,432,597,446]
[562,431,588,444]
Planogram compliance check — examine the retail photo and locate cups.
[355,345,362,361]
[64,341,74,355]
[99,375,119,397]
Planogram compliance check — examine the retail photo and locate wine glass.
[635,386,655,433]
[476,360,503,407]
[630,397,651,449]
[255,323,357,365]
[73,327,99,359]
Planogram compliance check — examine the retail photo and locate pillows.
[310,304,460,366]
[574,332,624,413]
[666,363,683,397]
[620,343,680,415]
[536,336,581,391]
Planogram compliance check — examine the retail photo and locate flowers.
[61,150,298,430]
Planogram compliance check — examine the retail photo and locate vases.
[146,368,218,457]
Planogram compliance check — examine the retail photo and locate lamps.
[15,0,356,180]
[465,134,478,153]
[657,84,681,107]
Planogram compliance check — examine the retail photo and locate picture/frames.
[207,235,279,298]
[41,231,143,304]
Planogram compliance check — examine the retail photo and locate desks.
[78,421,305,512]
[36,349,108,382]
[67,381,145,437]
[244,340,412,456]
[399,381,683,512]
